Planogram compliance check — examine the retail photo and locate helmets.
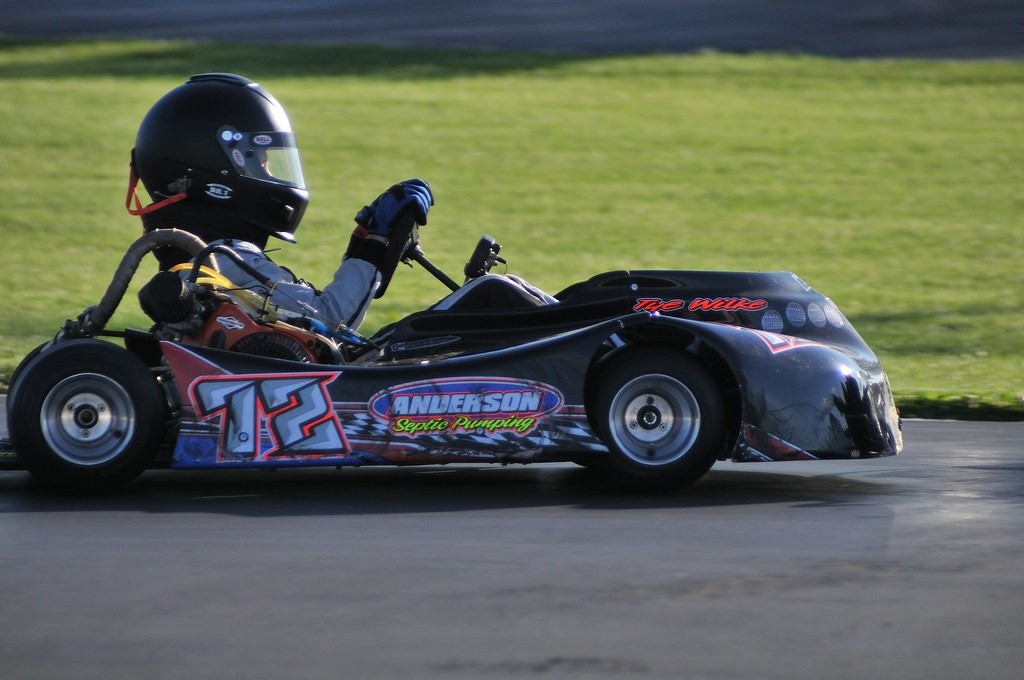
[129,73,311,245]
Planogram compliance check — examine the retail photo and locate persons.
[126,73,560,348]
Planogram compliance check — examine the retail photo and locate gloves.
[352,177,435,244]
